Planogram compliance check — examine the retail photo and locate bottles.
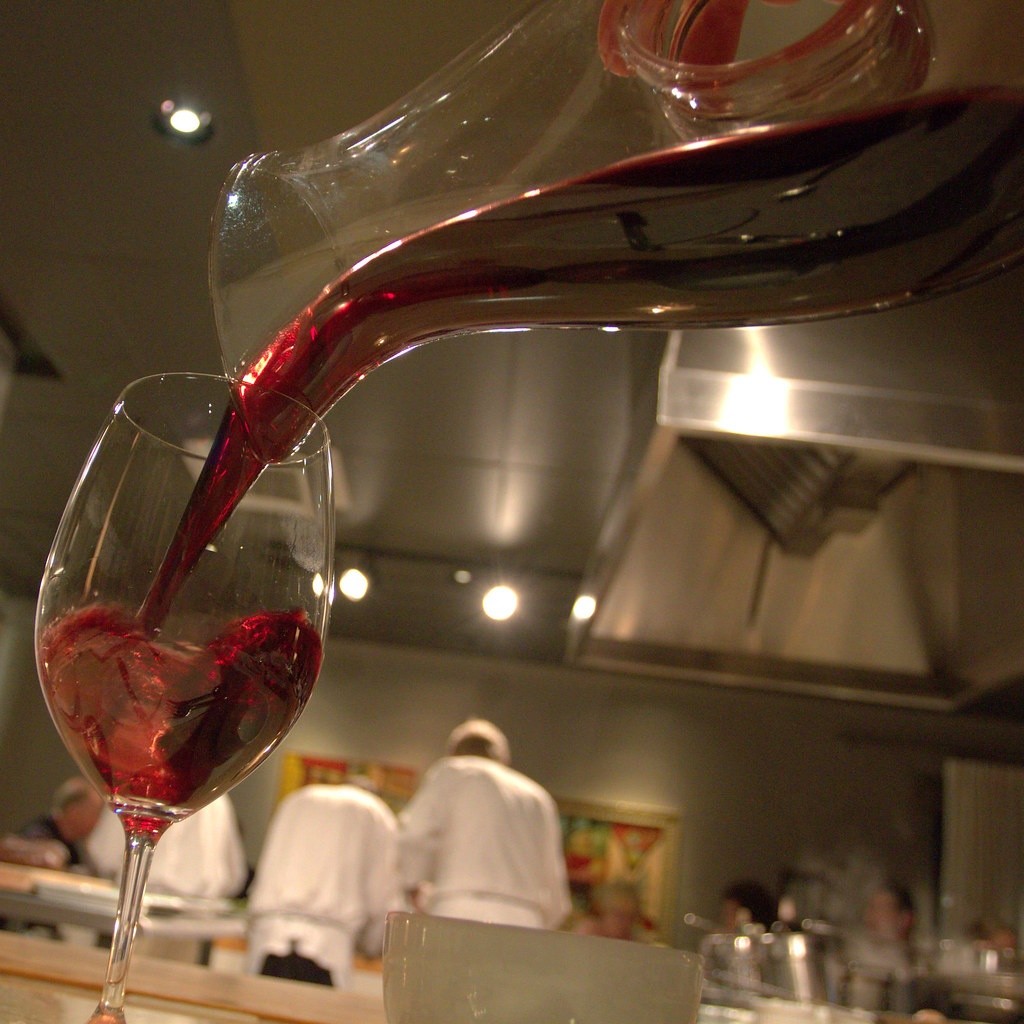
[208,2,1024,459]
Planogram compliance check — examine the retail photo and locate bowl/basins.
[381,909,707,1024]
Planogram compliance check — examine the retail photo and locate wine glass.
[34,371,333,1024]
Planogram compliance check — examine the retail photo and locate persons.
[82,787,249,969]
[566,868,1023,1023]
[400,717,580,933]
[254,771,411,990]
[1,776,107,950]
